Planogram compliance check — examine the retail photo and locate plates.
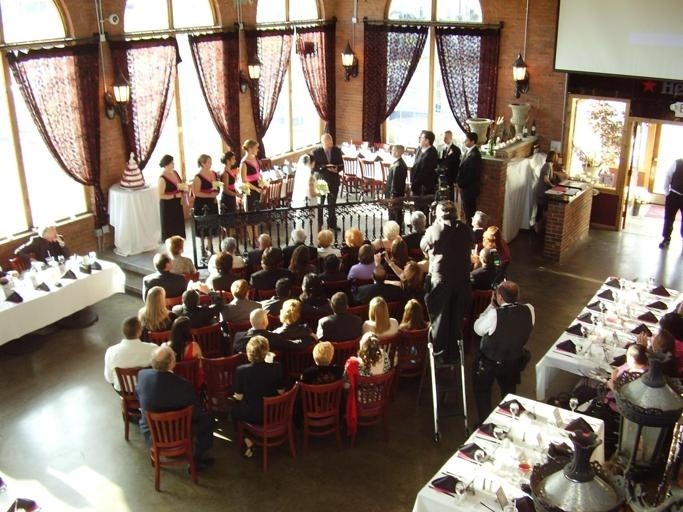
[550,275,678,367]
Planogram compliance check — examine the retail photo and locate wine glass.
[432,396,579,511]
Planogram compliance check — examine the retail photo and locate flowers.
[314,179,331,195]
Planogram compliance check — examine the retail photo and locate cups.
[0,250,96,298]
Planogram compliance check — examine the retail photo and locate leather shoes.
[243,445,260,459]
[187,456,215,474]
[330,225,341,231]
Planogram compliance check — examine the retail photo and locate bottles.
[263,160,298,181]
[347,144,416,164]
[523,122,528,138]
[488,136,494,157]
[531,118,536,136]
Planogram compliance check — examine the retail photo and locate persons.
[531,150,556,234]
[102,200,537,474]
[15,223,65,269]
[658,158,683,248]
[553,154,566,184]
[382,131,484,236]
[547,302,683,450]
[157,139,271,260]
[0,270,19,285]
[287,134,345,245]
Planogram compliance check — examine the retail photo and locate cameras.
[380,253,385,258]
[490,282,499,291]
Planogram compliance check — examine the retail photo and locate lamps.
[612,349,683,507]
[240,46,262,94]
[105,63,132,125]
[512,53,529,98]
[529,430,628,512]
[341,40,358,81]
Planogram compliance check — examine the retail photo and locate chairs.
[259,160,297,208]
[339,140,416,202]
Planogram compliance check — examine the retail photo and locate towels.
[431,399,596,512]
[555,279,672,368]
[0,251,102,304]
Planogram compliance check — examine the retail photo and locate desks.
[411,392,605,512]
[0,254,126,355]
[107,182,162,257]
[535,274,683,402]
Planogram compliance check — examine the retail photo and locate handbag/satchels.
[471,351,499,377]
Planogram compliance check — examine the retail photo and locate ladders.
[427,329,469,442]
